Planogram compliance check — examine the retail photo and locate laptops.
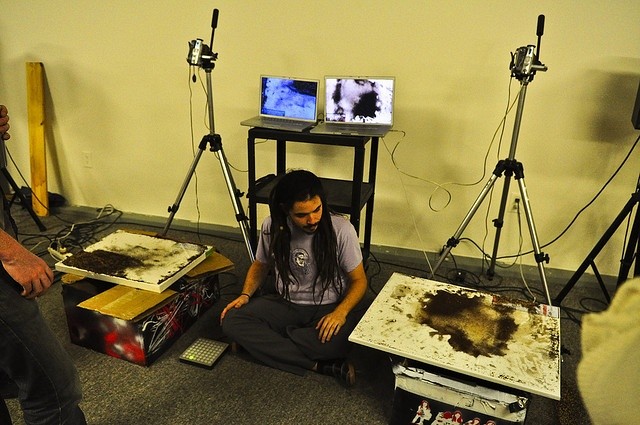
[240,74,320,131]
[310,75,396,138]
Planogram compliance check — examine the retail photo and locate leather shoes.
[330,360,358,389]
[232,341,245,353]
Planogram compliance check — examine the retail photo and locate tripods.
[555,86,640,308]
[0,165,47,234]
[426,15,554,307]
[161,7,255,264]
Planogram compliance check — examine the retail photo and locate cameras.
[185,38,219,74]
[509,43,548,86]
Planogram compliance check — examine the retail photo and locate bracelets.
[240,292,251,298]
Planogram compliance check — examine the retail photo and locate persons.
[431,410,497,425]
[411,400,432,425]
[220,170,368,385]
[294,252,307,276]
[1,103,88,424]
